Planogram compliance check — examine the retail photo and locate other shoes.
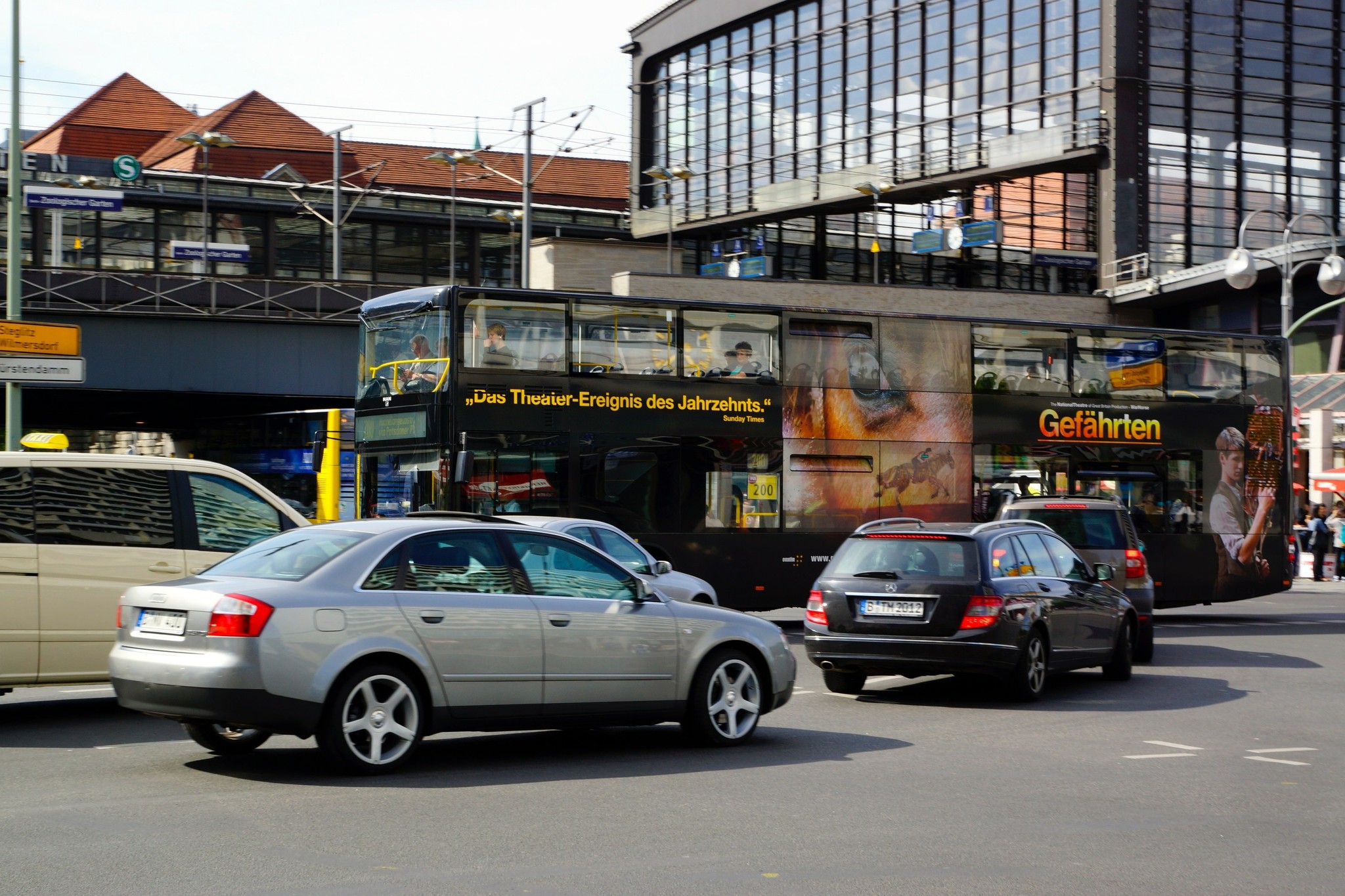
[1314,577,1329,581]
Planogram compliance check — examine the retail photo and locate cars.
[107,508,799,776]
[433,515,721,607]
[801,516,1143,703]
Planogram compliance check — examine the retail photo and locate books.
[389,366,405,376]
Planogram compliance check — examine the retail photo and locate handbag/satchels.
[1308,531,1328,547]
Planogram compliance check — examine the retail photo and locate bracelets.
[420,375,422,379]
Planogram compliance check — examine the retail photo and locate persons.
[1206,428,1277,599]
[481,323,515,372]
[731,485,778,528]
[721,348,738,367]
[723,342,752,382]
[1306,499,1345,581]
[496,483,522,512]
[396,335,449,394]
[1132,491,1191,534]
[413,542,448,591]
[1074,478,1122,505]
[1026,366,1040,392]
[1015,476,1030,498]
[706,505,724,527]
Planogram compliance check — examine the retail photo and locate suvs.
[992,489,1155,665]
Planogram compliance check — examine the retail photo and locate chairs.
[434,548,470,581]
[536,353,1103,393]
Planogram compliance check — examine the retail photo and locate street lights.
[853,180,898,284]
[423,149,484,286]
[1223,207,1345,344]
[641,165,699,274]
[484,207,538,287]
[176,130,238,281]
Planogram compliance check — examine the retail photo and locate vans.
[0,447,343,696]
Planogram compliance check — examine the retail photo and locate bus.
[311,282,1298,615]
